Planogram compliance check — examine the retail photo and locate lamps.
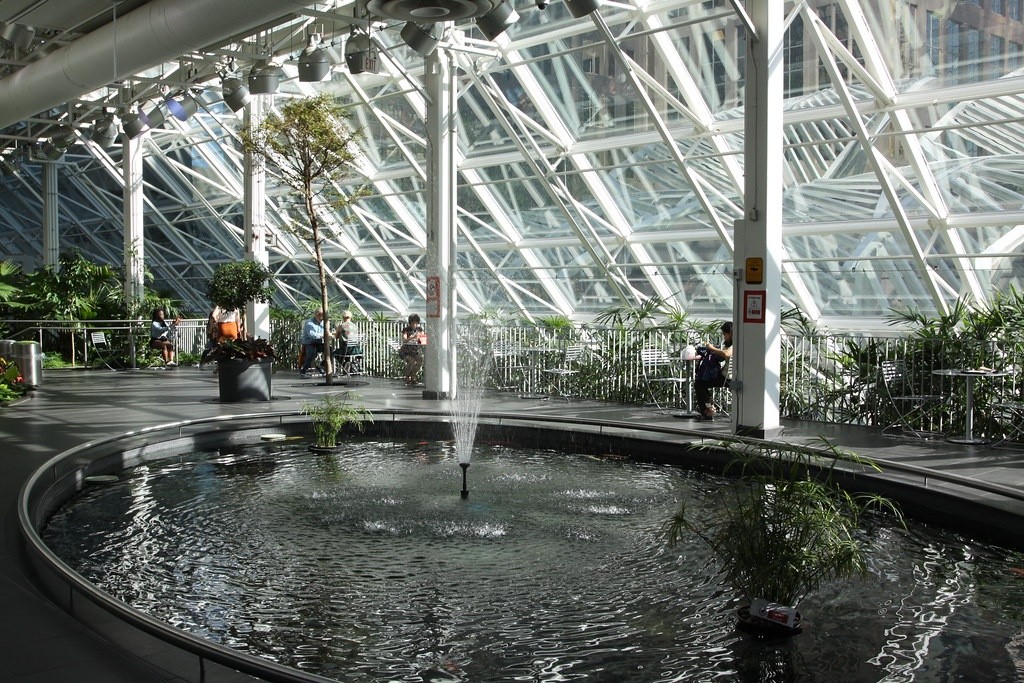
[1,0,600,179]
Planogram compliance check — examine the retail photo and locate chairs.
[456,340,466,373]
[709,355,731,420]
[991,401,1024,451]
[641,349,686,415]
[388,339,401,382]
[145,330,166,368]
[882,360,948,438]
[540,346,581,403]
[296,333,321,376]
[91,332,124,372]
[335,334,365,378]
[492,339,528,397]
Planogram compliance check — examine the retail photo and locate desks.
[403,342,426,387]
[114,334,149,370]
[508,347,556,399]
[932,369,1009,445]
[669,357,701,417]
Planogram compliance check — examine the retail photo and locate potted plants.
[663,432,908,629]
[301,389,373,454]
[205,258,278,402]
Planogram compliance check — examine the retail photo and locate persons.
[693,322,734,421]
[299,309,332,376]
[398,313,425,385]
[316,310,360,374]
[149,309,183,366]
[195,303,240,367]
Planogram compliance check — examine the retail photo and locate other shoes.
[406,376,412,383]
[166,365,172,369]
[167,360,177,366]
[695,415,714,421]
[316,363,324,374]
[411,376,418,383]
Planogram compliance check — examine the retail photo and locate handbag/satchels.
[695,357,721,380]
